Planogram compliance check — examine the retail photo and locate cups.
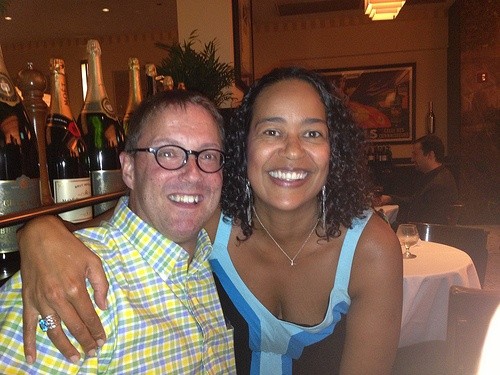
[371,186,382,207]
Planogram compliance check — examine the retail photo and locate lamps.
[364,0,406,21]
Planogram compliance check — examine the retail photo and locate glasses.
[127,145,230,173]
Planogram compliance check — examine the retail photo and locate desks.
[398,239,481,349]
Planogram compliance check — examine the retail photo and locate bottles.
[45,57,93,225]
[0,50,40,287]
[367,144,392,167]
[77,39,127,217]
[427,101,434,134]
[122,57,186,135]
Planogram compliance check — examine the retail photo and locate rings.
[37,315,61,331]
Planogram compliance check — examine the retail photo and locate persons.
[16,66,401,375]
[374,134,460,226]
[0,90,240,375]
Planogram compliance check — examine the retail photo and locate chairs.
[416,222,490,289]
[394,286,500,375]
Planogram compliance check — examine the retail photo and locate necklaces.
[252,203,319,267]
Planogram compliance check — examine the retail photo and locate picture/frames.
[314,63,417,146]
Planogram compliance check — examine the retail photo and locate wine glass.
[396,224,419,259]
